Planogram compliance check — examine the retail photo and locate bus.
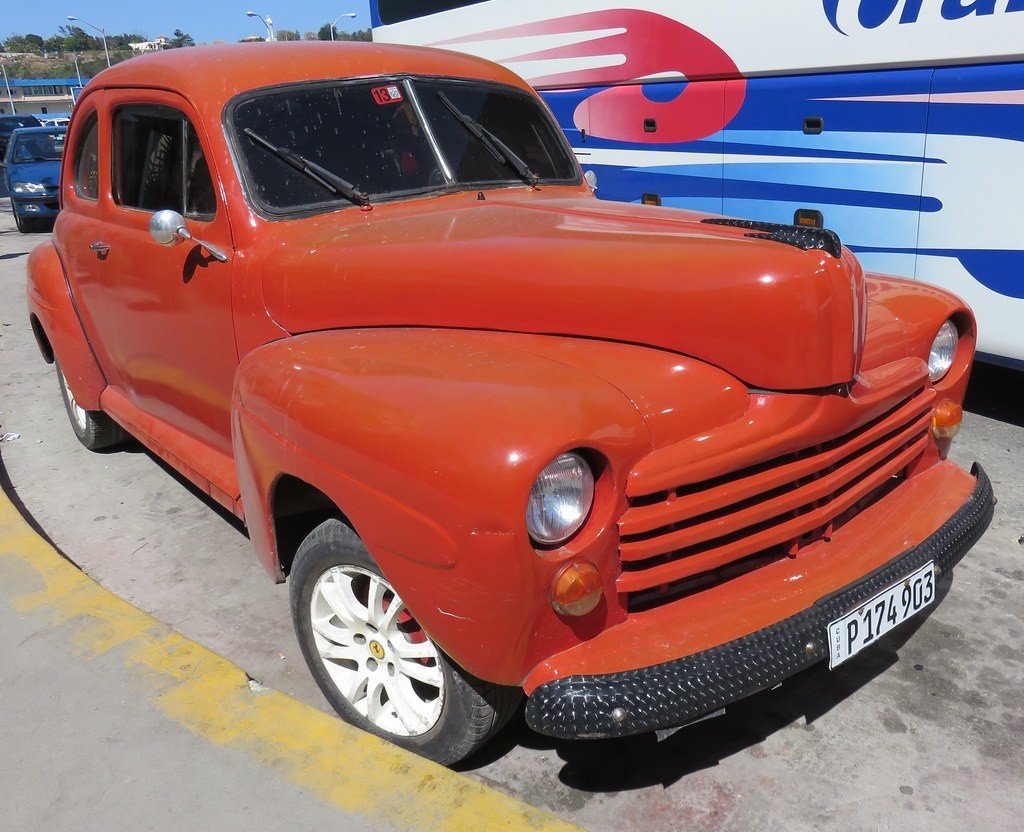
[367,0,1023,378]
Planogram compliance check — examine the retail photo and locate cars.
[24,36,1007,773]
[0,114,71,159]
[0,125,69,233]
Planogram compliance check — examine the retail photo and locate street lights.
[74,54,86,87]
[68,16,110,68]
[330,12,358,41]
[246,11,274,42]
